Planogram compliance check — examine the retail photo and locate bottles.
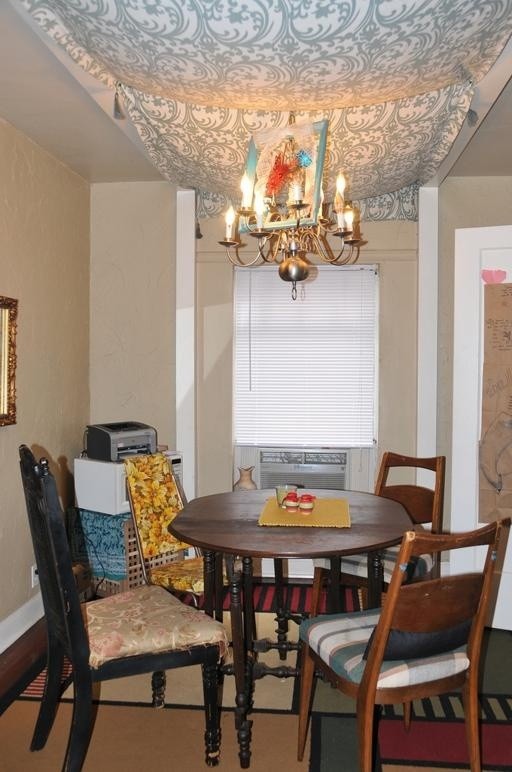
[285,493,314,515]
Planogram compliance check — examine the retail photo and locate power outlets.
[32,564,39,588]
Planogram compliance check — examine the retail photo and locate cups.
[275,485,297,507]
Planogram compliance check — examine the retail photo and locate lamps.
[218,118,361,301]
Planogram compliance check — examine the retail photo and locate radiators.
[256,449,350,490]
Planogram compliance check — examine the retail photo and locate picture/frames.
[0,295,18,427]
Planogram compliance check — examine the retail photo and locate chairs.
[297,516,512,772]
[124,452,254,609]
[310,451,446,617]
[18,444,231,772]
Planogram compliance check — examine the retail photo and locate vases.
[234,467,257,491]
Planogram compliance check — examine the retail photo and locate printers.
[86,421,157,462]
